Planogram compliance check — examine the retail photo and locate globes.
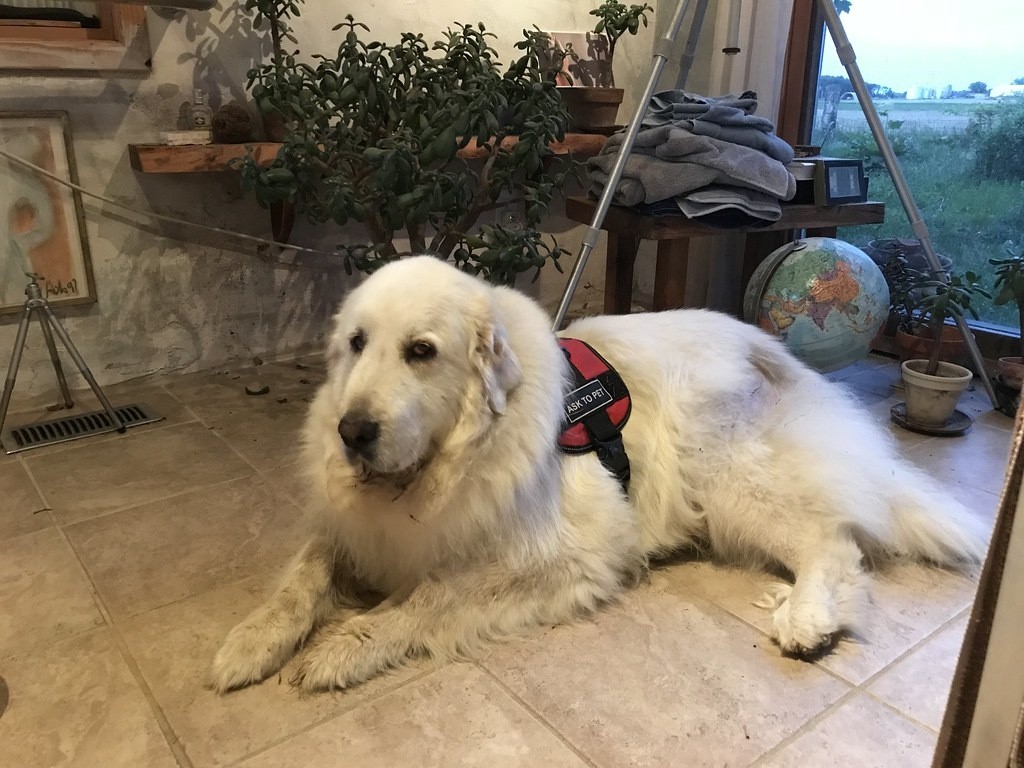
[739,237,891,374]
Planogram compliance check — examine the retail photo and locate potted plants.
[882,243,976,366]
[989,248,1024,417]
[901,272,992,427]
[245,0,316,143]
[556,0,655,133]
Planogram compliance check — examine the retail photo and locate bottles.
[186,88,216,143]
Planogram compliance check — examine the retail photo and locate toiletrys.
[186,88,215,131]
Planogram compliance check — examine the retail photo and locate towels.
[585,81,802,228]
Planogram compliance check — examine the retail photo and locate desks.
[566,196,885,317]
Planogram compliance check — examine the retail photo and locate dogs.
[200,254,995,696]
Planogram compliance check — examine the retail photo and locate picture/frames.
[0,110,100,318]
